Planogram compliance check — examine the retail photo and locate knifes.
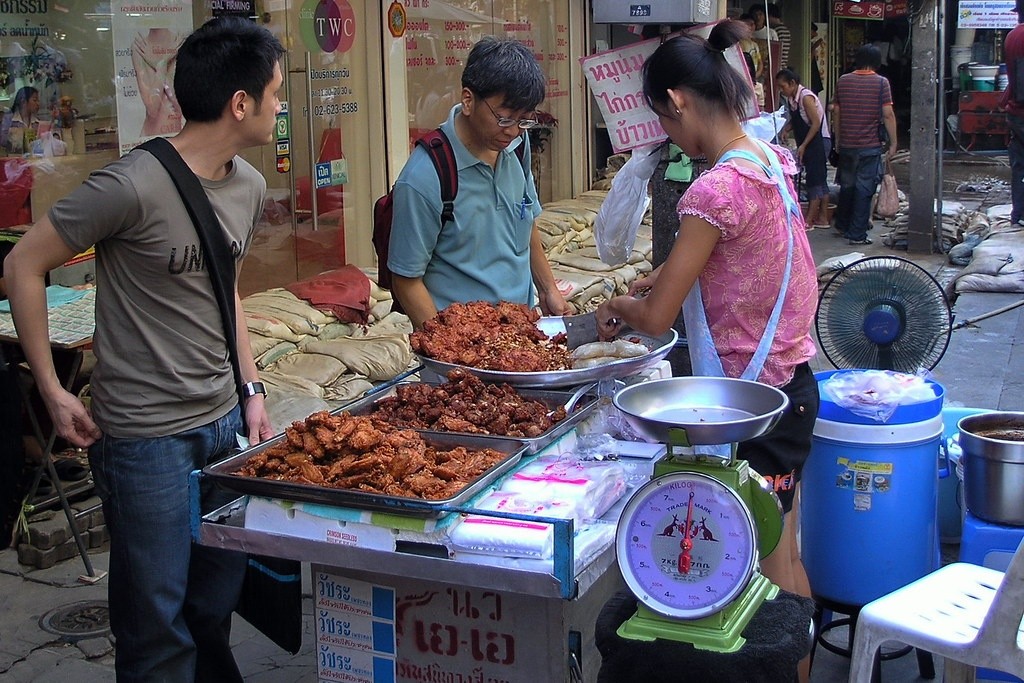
[560,286,653,352]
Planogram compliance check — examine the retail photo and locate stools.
[596,588,816,683]
[957,507,1024,683]
[809,589,935,683]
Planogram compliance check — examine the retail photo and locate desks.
[0,285,97,577]
[190,441,695,683]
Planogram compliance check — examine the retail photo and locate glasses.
[484,99,538,128]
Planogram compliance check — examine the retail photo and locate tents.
[405,0,508,24]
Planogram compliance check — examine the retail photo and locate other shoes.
[835,223,848,237]
[849,238,873,244]
[53,460,87,481]
[805,223,814,231]
[1016,217,1024,226]
[18,463,52,493]
[814,224,832,228]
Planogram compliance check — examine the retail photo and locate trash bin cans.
[798,368,947,607]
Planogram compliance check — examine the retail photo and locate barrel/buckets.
[800,368,953,608]
[972,77,998,91]
[957,410,1024,531]
[959,62,979,92]
[938,408,998,545]
[950,28,976,77]
[998,74,1009,91]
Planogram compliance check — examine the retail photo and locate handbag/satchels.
[592,144,661,267]
[878,124,887,141]
[877,159,899,218]
[234,553,302,656]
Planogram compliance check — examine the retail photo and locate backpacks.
[372,128,525,290]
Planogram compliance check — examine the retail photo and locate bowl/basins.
[968,65,999,77]
[999,63,1007,74]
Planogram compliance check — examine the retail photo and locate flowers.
[527,110,558,153]
[51,97,77,129]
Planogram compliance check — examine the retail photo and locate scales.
[611,376,789,653]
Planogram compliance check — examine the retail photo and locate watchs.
[242,382,267,399]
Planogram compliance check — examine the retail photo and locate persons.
[131,28,186,138]
[737,5,912,245]
[4,17,286,683]
[594,18,819,683]
[416,49,464,129]
[0,86,42,156]
[1,193,87,496]
[388,33,572,382]
[1004,0,1024,230]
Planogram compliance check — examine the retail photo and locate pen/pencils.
[520,197,526,219]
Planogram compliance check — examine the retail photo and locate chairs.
[849,538,1024,683]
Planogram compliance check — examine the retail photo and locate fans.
[815,255,956,375]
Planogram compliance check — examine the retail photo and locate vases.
[60,128,74,156]
[531,151,541,201]
